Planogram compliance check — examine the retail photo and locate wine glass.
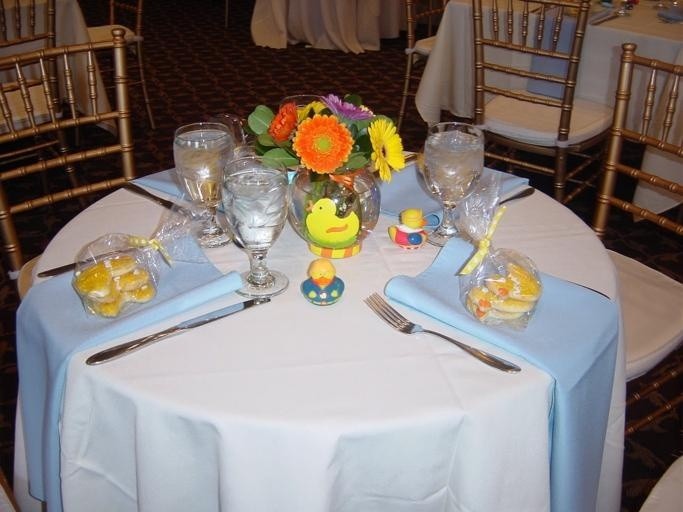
[172,123,233,247]
[421,121,486,248]
[218,155,292,300]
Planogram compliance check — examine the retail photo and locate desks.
[415,0,683,222]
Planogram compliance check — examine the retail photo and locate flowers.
[242,93,405,182]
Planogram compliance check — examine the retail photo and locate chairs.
[473,0,614,206]
[1,1,136,277]
[86,1,156,130]
[396,0,447,133]
[592,42,683,437]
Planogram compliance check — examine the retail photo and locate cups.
[278,95,321,110]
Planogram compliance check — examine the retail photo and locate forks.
[362,292,523,374]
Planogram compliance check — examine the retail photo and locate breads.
[74,255,155,317]
[466,263,541,320]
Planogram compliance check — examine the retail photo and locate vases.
[288,168,381,258]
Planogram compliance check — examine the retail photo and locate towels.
[378,160,530,228]
[383,237,618,512]
[16,233,243,512]
[134,168,225,214]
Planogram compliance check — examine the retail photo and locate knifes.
[119,181,206,221]
[591,14,620,26]
[85,297,272,366]
